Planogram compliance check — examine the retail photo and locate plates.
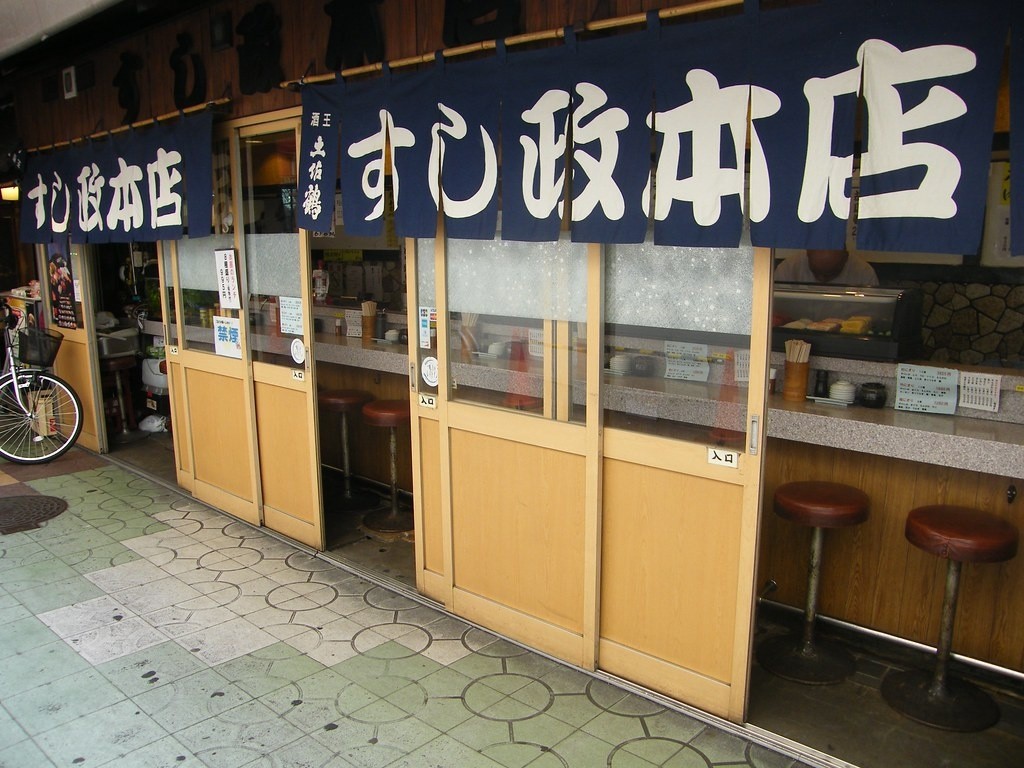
[829,380,856,403]
[487,341,505,357]
[384,330,399,342]
[608,356,633,374]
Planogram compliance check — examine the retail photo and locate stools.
[358,398,415,533]
[879,503,1019,733]
[752,480,872,688]
[104,354,152,443]
[318,387,384,516]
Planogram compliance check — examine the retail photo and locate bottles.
[859,383,887,408]
[250,313,262,326]
[199,303,239,328]
[399,329,408,345]
[812,369,829,399]
[313,318,322,332]
[335,319,342,336]
[633,356,653,377]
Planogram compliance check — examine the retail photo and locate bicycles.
[1,298,83,466]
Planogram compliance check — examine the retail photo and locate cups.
[461,325,478,355]
[361,315,376,341]
[576,338,587,372]
[509,342,524,364]
[768,378,776,395]
[782,359,810,401]
[376,313,387,339]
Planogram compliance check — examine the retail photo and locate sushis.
[771,311,878,334]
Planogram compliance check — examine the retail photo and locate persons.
[772,249,886,288]
[118,256,346,308]
